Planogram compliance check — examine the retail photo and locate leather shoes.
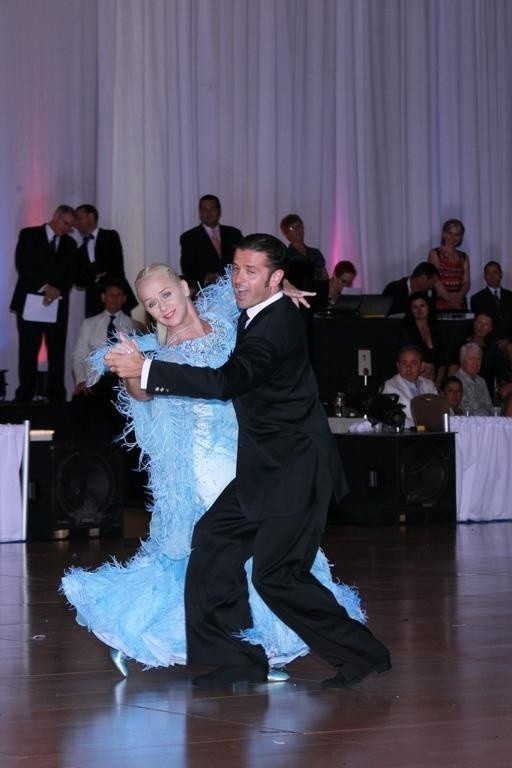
[192,670,269,689]
[322,651,392,691]
[109,646,129,677]
[267,669,292,683]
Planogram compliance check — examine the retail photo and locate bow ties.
[84,235,94,243]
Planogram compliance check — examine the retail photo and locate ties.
[107,316,117,345]
[44,234,57,255]
[493,290,501,307]
[237,308,249,340]
[213,229,222,261]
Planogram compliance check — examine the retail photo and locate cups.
[333,391,345,417]
[490,406,502,416]
[464,406,474,416]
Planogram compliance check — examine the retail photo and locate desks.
[1,296,511,543]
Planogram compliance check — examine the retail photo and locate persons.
[379,346,438,418]
[56,262,368,681]
[109,232,391,685]
[179,195,246,291]
[8,205,78,407]
[384,219,511,417]
[72,205,141,321]
[280,213,330,311]
[72,277,143,394]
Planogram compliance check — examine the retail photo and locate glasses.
[337,275,351,288]
[287,222,303,234]
